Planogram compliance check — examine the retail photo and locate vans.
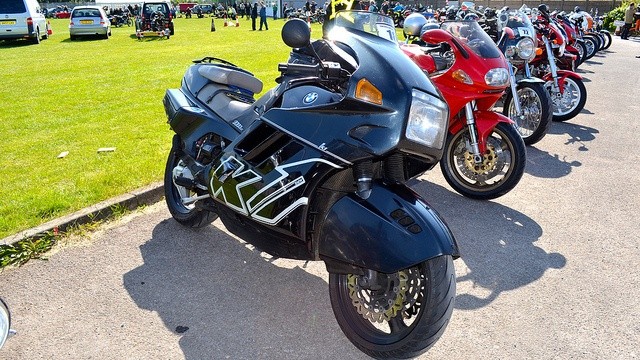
[191,3,212,14]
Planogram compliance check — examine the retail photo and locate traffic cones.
[235,15,240,27]
[223,15,227,27]
[211,18,215,31]
[47,22,52,35]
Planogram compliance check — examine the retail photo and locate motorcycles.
[110,12,133,27]
[286,2,482,26]
[614,14,639,35]
[164,10,460,360]
[482,5,612,144]
[398,20,525,199]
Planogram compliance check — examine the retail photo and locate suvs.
[136,3,174,38]
[0,0,48,43]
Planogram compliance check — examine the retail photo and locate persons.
[112,0,140,27]
[282,1,444,26]
[44,0,109,32]
[168,2,279,31]
[621,2,640,40]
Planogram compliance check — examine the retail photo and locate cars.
[70,6,111,40]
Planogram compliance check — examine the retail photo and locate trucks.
[179,3,196,13]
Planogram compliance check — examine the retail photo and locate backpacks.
[372,5,377,12]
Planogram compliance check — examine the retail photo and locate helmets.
[574,6,580,12]
[420,23,440,40]
[463,13,478,21]
[275,40,359,83]
[538,4,550,13]
[403,12,427,36]
